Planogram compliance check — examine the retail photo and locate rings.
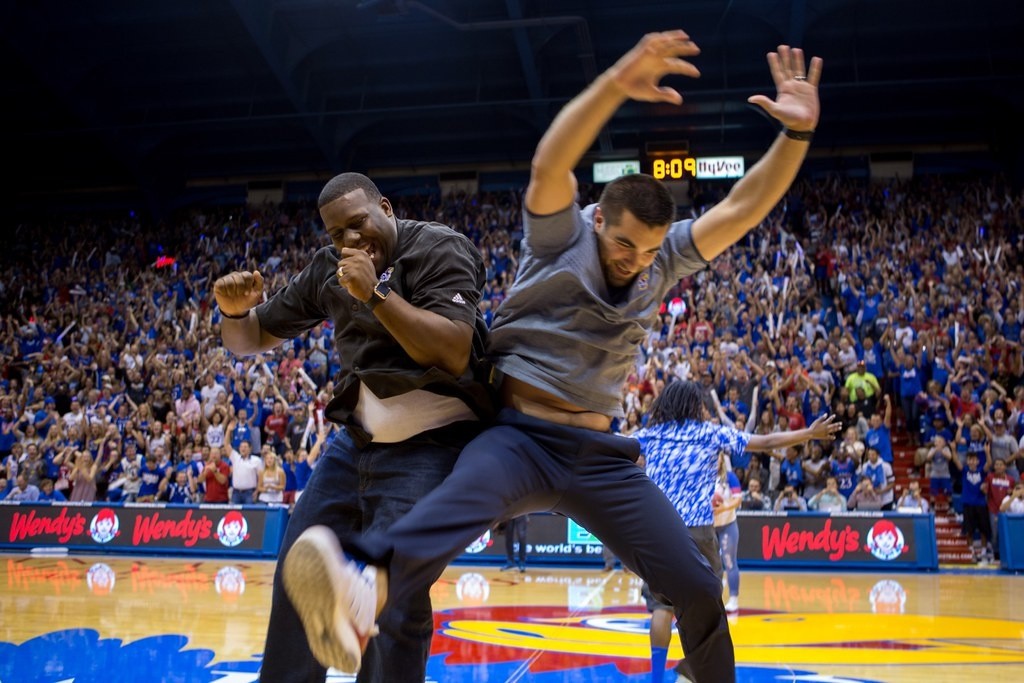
[339,266,343,276]
[795,76,807,80]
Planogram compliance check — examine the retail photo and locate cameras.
[783,492,792,497]
[908,489,914,494]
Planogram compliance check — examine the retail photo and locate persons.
[610,174,1024,565]
[628,380,843,683]
[282,29,822,683]
[0,185,522,502]
[213,172,489,683]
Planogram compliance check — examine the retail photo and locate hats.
[993,419,1004,426]
[103,383,113,391]
[857,361,866,366]
[101,375,111,382]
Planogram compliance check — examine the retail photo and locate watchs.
[365,282,391,311]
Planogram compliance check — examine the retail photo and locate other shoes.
[281,526,379,674]
[725,598,738,611]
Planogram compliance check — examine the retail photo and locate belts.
[233,488,255,494]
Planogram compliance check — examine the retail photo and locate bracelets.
[219,307,250,319]
[781,126,815,140]
